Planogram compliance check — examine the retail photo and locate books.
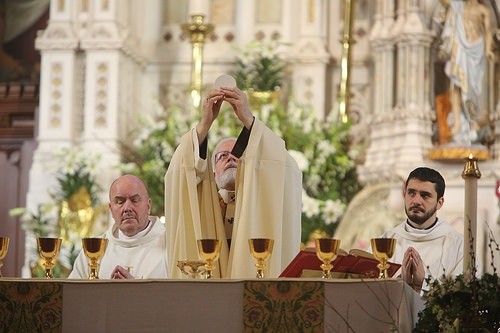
[278,247,402,278]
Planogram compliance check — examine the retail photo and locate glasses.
[215,150,231,165]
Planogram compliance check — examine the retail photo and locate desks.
[0,277,422,333]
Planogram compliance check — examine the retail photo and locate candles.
[461,156,482,275]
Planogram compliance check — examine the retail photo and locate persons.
[438,0,494,136]
[367,167,480,297]
[67,175,168,279]
[164,87,302,278]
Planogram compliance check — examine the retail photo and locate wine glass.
[0,236,11,278]
[314,238,340,280]
[197,239,224,280]
[248,238,275,278]
[37,237,62,278]
[82,237,109,280]
[370,238,397,279]
